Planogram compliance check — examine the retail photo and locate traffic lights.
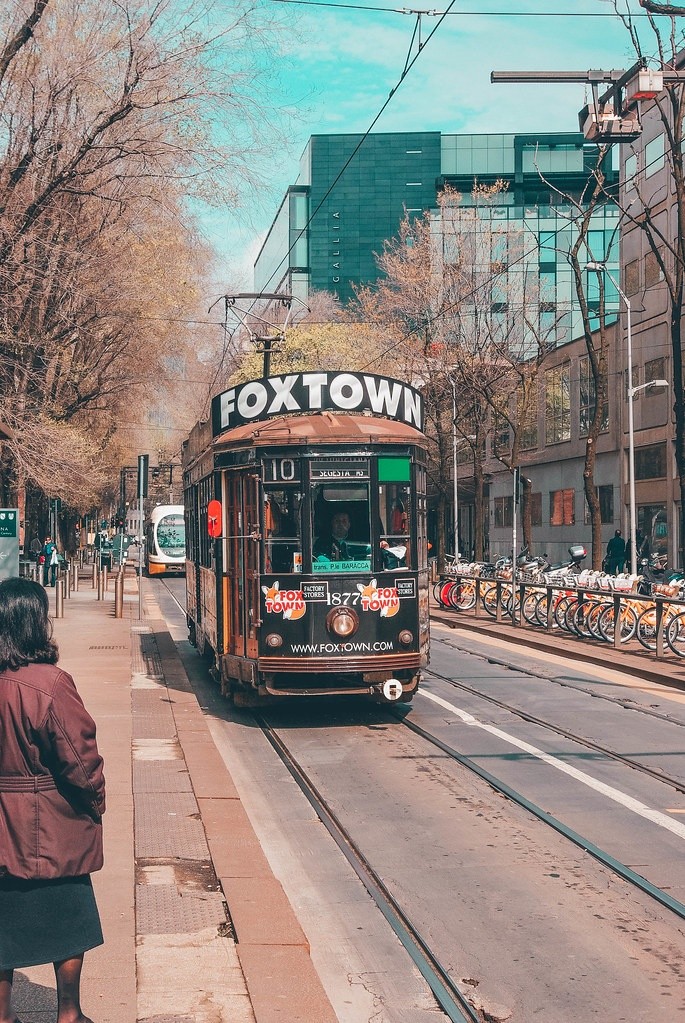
[119,518,124,527]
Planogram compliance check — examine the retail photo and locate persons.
[41,537,56,586]
[134,536,138,546]
[625,529,649,574]
[0,578,105,1023]
[391,495,409,535]
[313,512,388,561]
[607,530,625,574]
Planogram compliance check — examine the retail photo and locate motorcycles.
[518,540,588,574]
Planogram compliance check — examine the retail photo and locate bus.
[178,369,430,705]
[144,504,185,578]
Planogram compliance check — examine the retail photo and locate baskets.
[444,562,679,601]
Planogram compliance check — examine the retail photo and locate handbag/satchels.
[50,549,58,566]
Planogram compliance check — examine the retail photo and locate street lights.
[453,435,476,561]
[584,261,669,578]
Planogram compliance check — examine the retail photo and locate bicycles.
[433,554,685,659]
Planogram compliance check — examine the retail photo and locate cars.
[130,535,136,545]
